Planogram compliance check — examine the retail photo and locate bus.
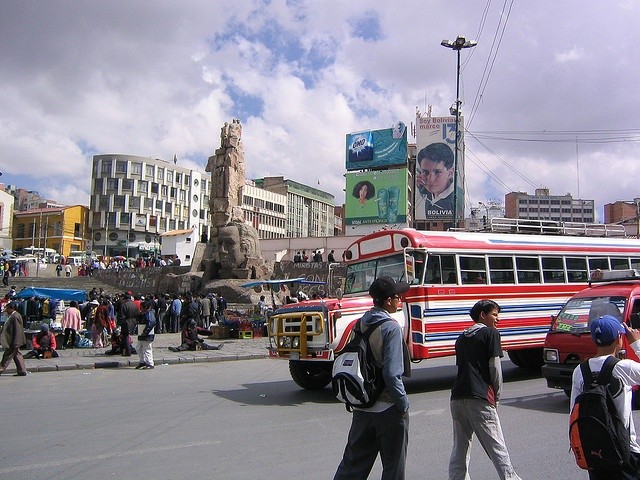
[7,255,48,271]
[65,250,98,266]
[266,217,640,390]
[21,248,56,265]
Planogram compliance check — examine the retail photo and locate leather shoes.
[13,373,26,376]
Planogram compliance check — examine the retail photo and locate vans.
[49,253,64,263]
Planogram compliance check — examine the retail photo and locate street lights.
[440,33,480,230]
[478,199,495,229]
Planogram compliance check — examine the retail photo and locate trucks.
[543,272,640,408]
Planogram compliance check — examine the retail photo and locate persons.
[1,242,229,376]
[301,250,308,262]
[474,272,486,284]
[416,142,464,220]
[309,250,316,262]
[332,276,410,480]
[327,249,336,262]
[257,284,331,332]
[341,250,345,261]
[447,299,524,480]
[293,251,301,262]
[568,313,640,479]
[351,180,376,205]
[315,250,323,262]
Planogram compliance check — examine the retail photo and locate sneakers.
[216,342,223,349]
[143,364,154,369]
[135,362,146,369]
[168,347,178,352]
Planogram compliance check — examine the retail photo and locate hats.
[369,277,410,302]
[41,324,48,331]
[590,315,626,343]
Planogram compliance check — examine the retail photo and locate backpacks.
[569,356,630,470]
[332,318,391,412]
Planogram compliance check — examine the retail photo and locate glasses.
[392,296,402,299]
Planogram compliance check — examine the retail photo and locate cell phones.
[418,171,428,194]
